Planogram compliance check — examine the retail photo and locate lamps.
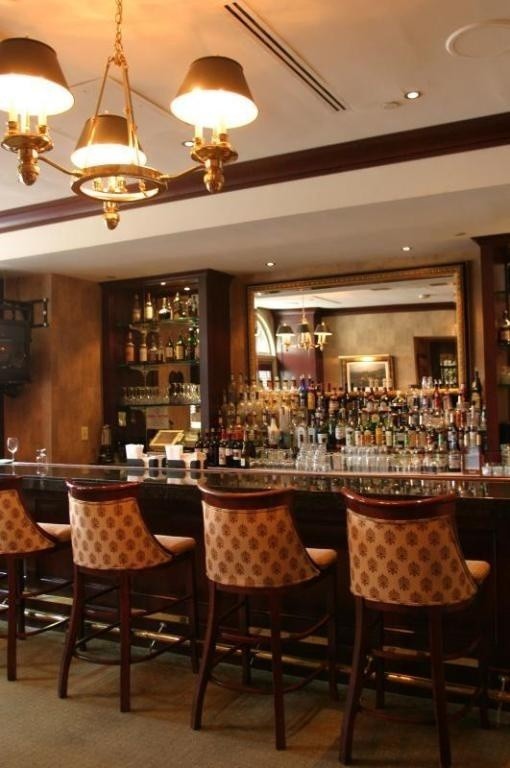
[273,306,334,354]
[0,1,263,232]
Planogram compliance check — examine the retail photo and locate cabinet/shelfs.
[97,265,232,463]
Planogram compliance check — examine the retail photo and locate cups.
[261,448,292,464]
[295,442,326,471]
[481,466,492,476]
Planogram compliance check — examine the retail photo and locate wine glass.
[173,385,201,404]
[7,437,18,463]
[122,387,167,404]
[35,449,47,464]
[422,377,431,389]
[341,447,390,471]
[392,448,438,472]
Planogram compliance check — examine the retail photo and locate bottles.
[164,337,174,363]
[158,341,164,363]
[186,330,195,358]
[209,431,216,457]
[194,431,202,452]
[217,371,489,498]
[145,291,154,322]
[175,335,185,360]
[190,296,197,316]
[139,331,148,365]
[149,344,158,364]
[124,331,136,366]
[202,430,209,455]
[173,290,182,320]
[158,296,172,321]
[131,293,142,322]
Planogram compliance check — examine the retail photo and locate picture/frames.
[338,354,396,391]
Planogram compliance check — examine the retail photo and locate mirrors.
[244,260,468,398]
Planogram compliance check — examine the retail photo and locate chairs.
[333,484,498,768]
[185,478,340,752]
[0,473,89,684]
[52,475,200,715]
